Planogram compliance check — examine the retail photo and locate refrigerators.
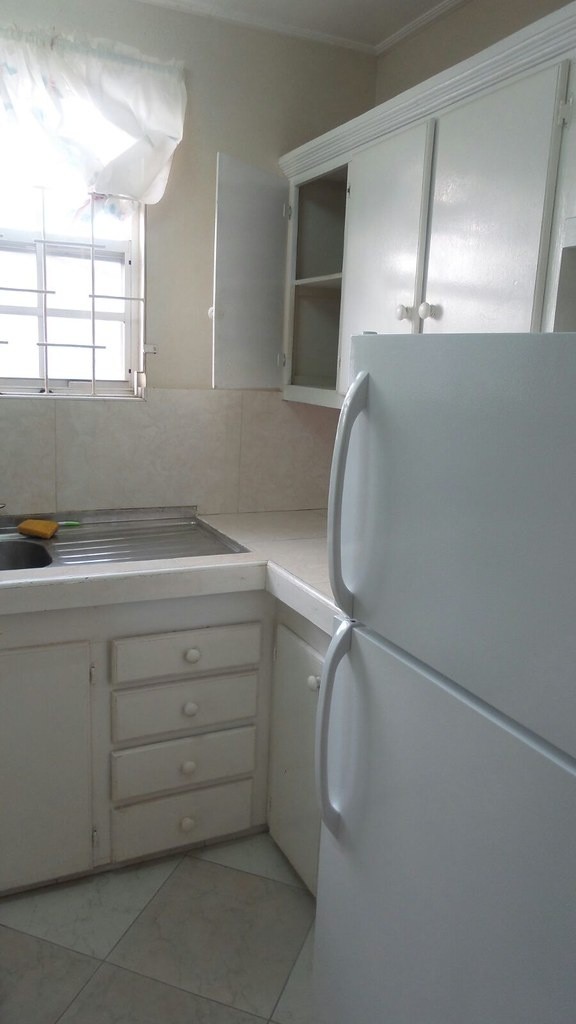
[315,333,576,1024]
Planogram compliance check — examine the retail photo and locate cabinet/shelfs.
[0,589,337,901]
[208,1,576,413]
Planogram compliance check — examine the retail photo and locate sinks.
[0,536,53,572]
